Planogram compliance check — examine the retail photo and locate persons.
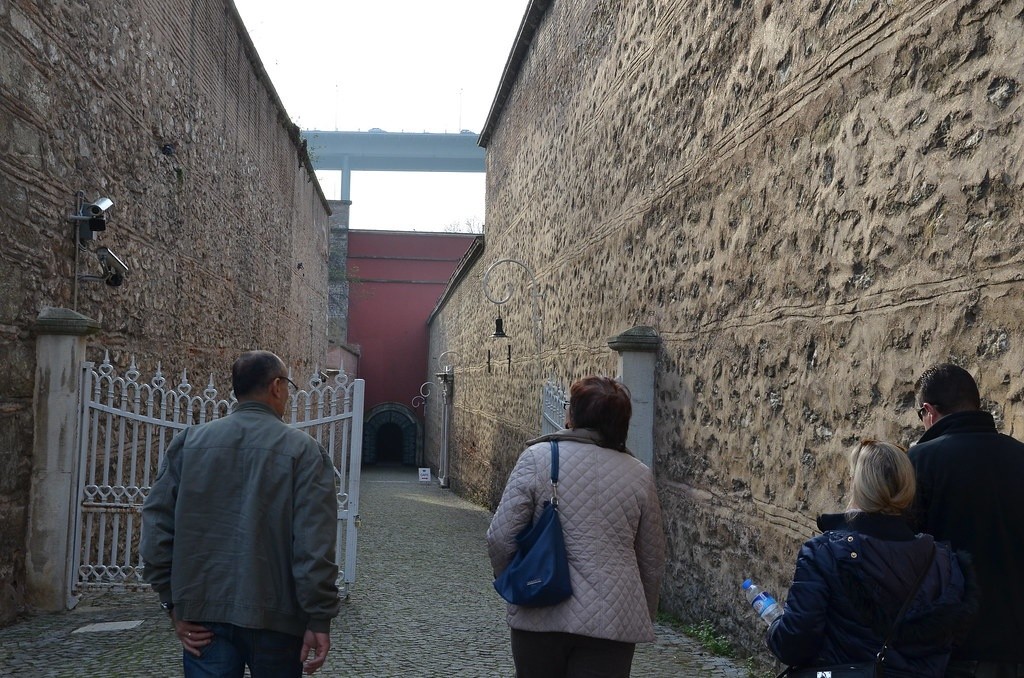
[764,361,1024,678]
[138,350,339,678]
[484,376,667,678]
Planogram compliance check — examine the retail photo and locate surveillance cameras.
[88,198,114,216]
[96,246,129,274]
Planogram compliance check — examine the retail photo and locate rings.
[187,632,193,639]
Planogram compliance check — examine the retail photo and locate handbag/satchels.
[493,439,576,608]
[775,662,880,678]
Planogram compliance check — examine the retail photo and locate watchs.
[160,603,175,614]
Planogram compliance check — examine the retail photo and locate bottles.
[742,579,784,627]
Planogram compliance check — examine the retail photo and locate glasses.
[851,438,908,489]
[563,400,571,411]
[273,376,300,395]
[916,405,937,422]
